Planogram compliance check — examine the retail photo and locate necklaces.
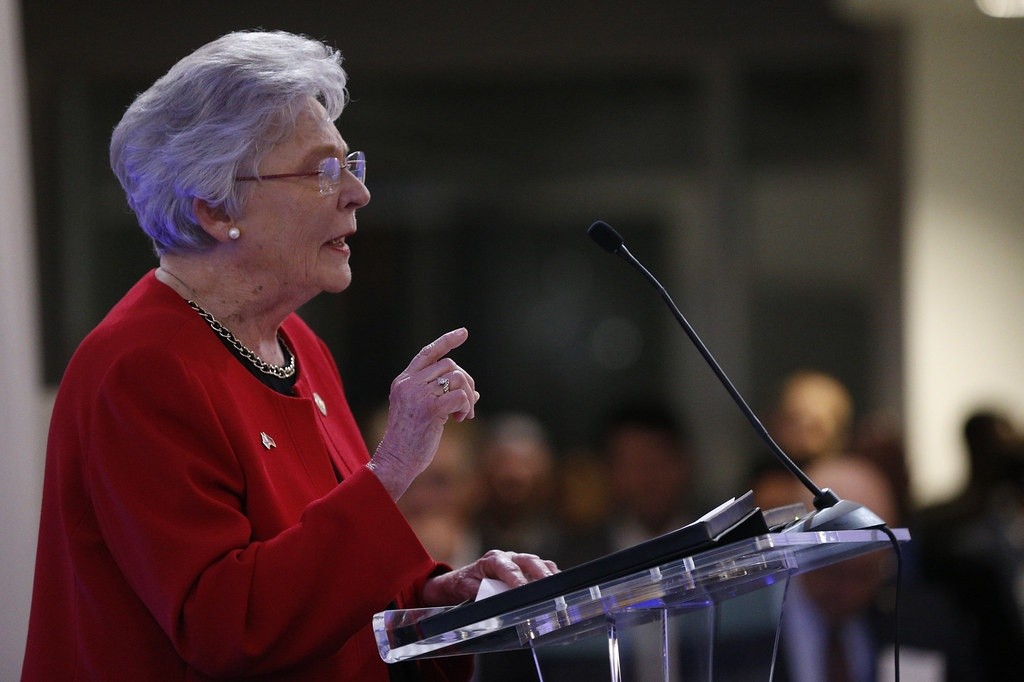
[188,298,295,377]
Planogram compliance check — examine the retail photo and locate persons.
[22,31,563,682]
[370,408,722,681]
[740,371,899,682]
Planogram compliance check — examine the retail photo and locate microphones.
[585,220,887,534]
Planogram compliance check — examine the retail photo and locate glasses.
[234,150,366,199]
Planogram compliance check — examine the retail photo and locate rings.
[437,377,450,393]
